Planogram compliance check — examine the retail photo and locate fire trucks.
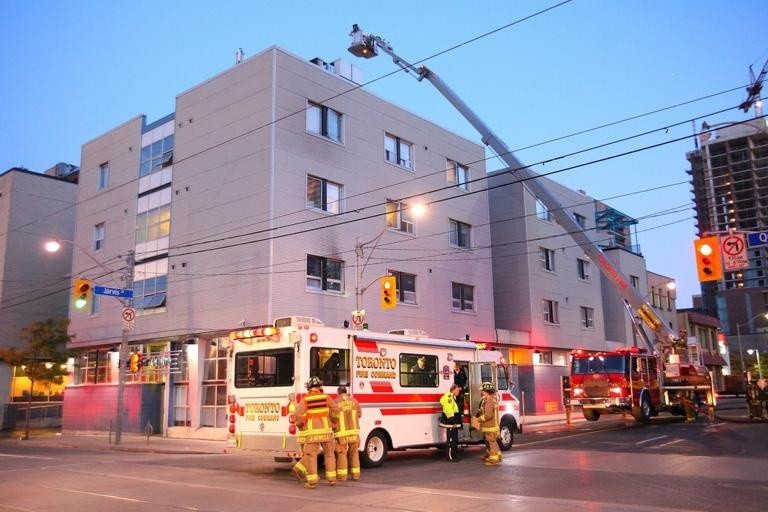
[348,24,718,423]
[224,315,524,468]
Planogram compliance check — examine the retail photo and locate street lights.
[698,120,768,143]
[355,201,426,311]
[45,239,136,445]
[736,313,768,392]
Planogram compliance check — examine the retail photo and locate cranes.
[739,54,768,119]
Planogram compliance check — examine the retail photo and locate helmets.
[307,376,322,386]
[482,382,493,391]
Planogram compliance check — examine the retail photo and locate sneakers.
[482,455,503,466]
[293,465,362,489]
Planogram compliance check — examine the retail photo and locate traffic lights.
[694,236,724,282]
[73,278,91,312]
[131,352,143,374]
[381,276,396,309]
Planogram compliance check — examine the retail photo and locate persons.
[328,384,362,481]
[473,381,493,459]
[478,382,503,465]
[452,362,467,417]
[440,383,460,462]
[682,378,768,428]
[289,375,336,488]
[407,354,435,387]
[323,352,340,385]
